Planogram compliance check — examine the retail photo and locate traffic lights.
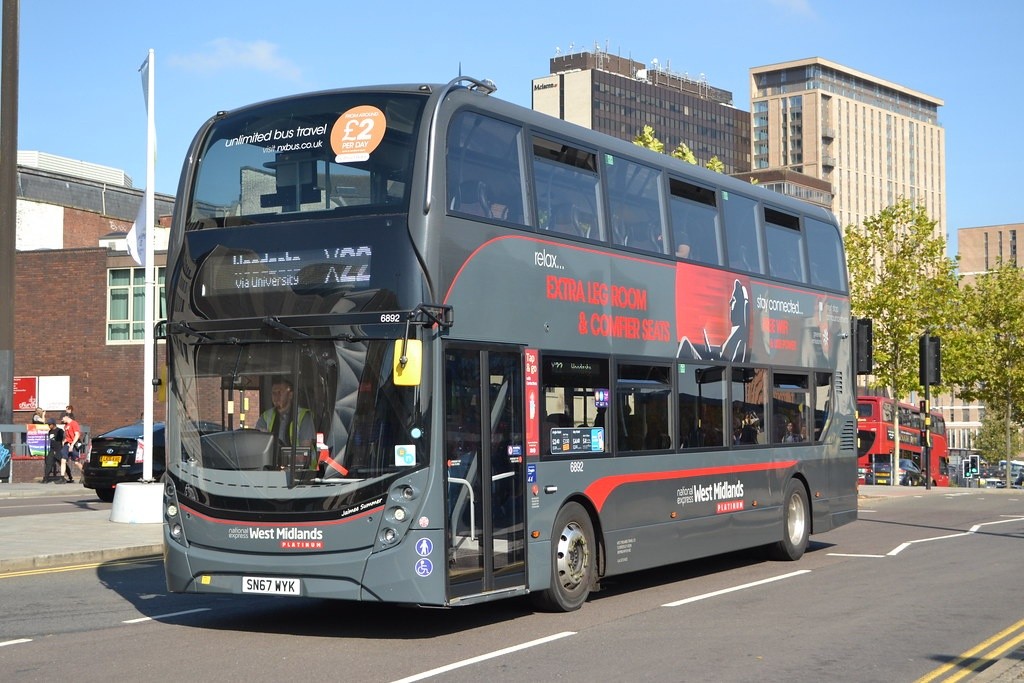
[963,459,972,478]
[969,455,979,475]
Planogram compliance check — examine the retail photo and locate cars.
[80,420,232,503]
[865,460,925,485]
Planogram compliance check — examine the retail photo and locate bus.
[152,77,857,612]
[857,396,950,487]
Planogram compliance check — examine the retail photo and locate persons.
[41,413,84,485]
[254,380,317,471]
[61,405,76,424]
[782,422,802,443]
[32,407,46,424]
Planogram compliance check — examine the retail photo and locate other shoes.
[55,478,66,484]
[79,474,84,483]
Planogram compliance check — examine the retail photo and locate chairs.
[545,413,823,454]
[448,181,822,288]
[310,386,340,447]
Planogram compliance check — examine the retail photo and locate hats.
[57,412,68,420]
[44,418,56,424]
